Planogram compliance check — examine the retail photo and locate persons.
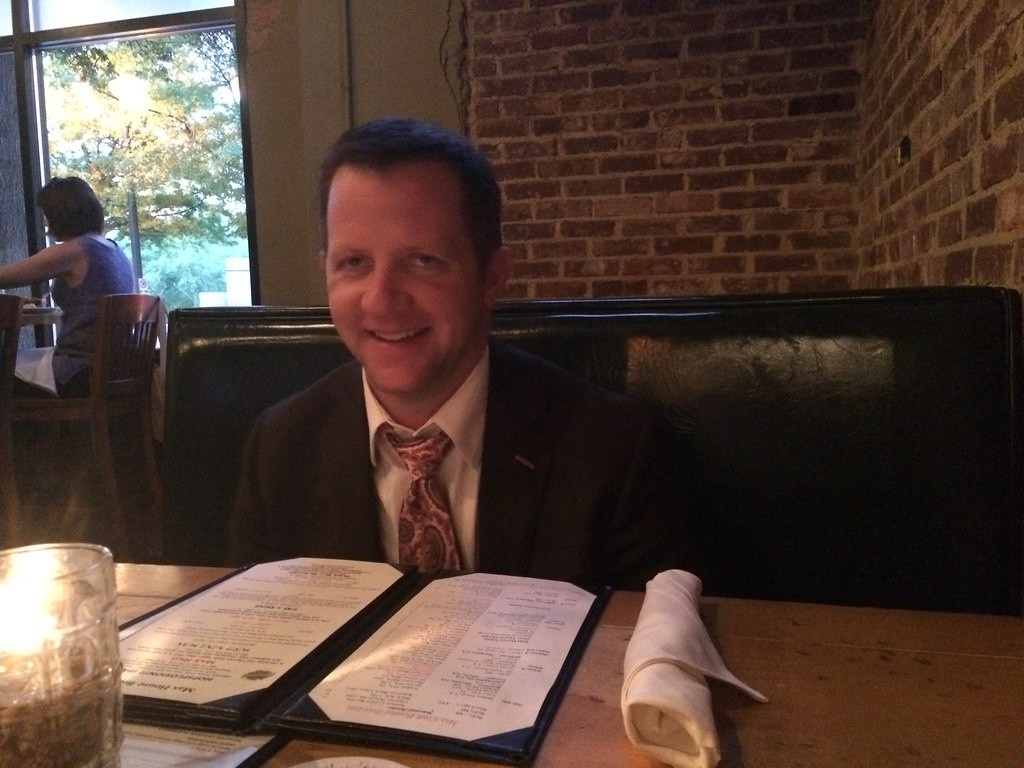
[0,178,137,398]
[226,119,704,592]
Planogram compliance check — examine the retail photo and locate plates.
[24,307,56,314]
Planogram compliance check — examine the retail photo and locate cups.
[0,543,125,767]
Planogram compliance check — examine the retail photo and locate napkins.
[621,569,770,768]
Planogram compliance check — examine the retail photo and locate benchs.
[164,286,1024,615]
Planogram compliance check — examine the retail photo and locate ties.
[380,422,462,573]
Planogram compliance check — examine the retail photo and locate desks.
[117,563,1024,768]
[23,310,62,325]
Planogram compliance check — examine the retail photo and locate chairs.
[0,294,25,547]
[12,293,168,559]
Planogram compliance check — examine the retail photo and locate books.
[118,557,616,767]
